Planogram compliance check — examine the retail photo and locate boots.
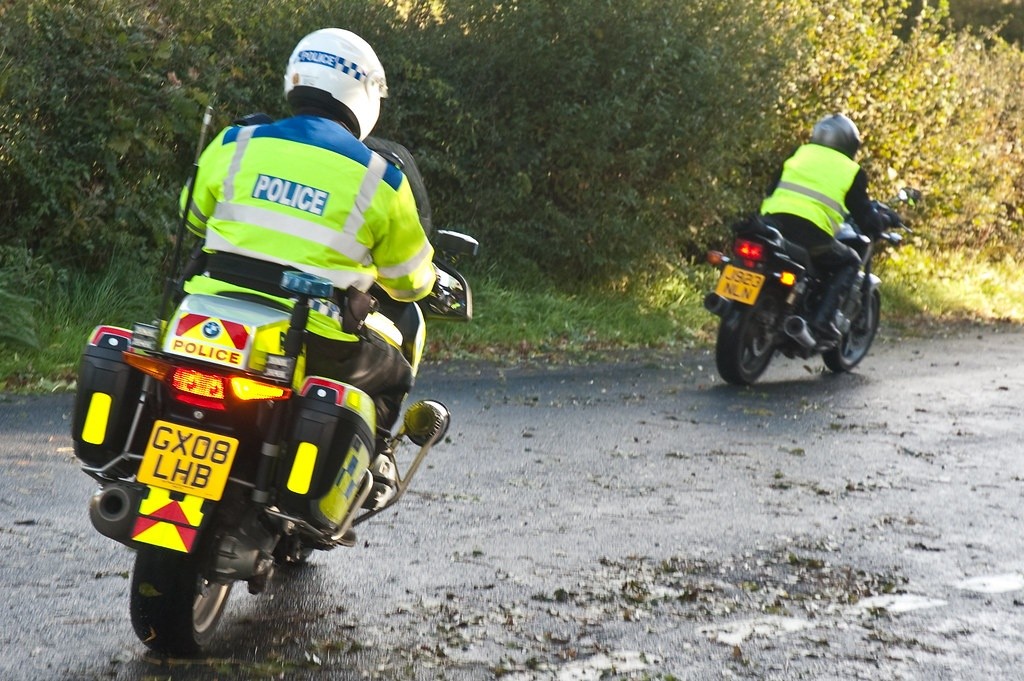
[808,266,856,340]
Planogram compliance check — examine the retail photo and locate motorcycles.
[68,140,483,655]
[703,173,926,387]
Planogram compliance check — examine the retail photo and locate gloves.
[881,209,899,228]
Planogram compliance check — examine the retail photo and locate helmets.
[812,114,860,160]
[282,28,389,143]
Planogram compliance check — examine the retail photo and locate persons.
[760,111,892,342]
[176,24,437,548]
